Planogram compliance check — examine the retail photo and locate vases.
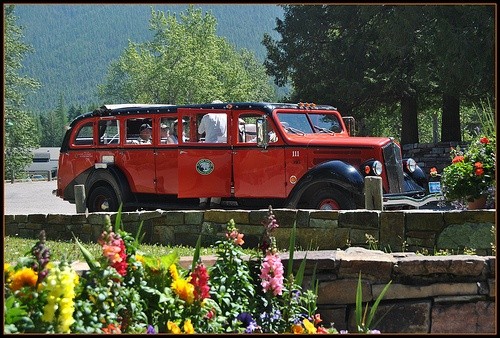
[465,195,487,209]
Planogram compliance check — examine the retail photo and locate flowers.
[430,137,495,204]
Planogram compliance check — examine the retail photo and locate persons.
[167,118,191,143]
[134,123,153,144]
[158,122,170,145]
[198,99,245,209]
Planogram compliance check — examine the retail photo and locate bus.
[54,100,427,213]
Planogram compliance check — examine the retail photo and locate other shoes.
[196,202,210,209]
[209,201,237,208]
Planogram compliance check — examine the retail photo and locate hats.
[173,118,185,124]
[139,124,152,131]
[212,100,223,104]
[161,123,169,129]
